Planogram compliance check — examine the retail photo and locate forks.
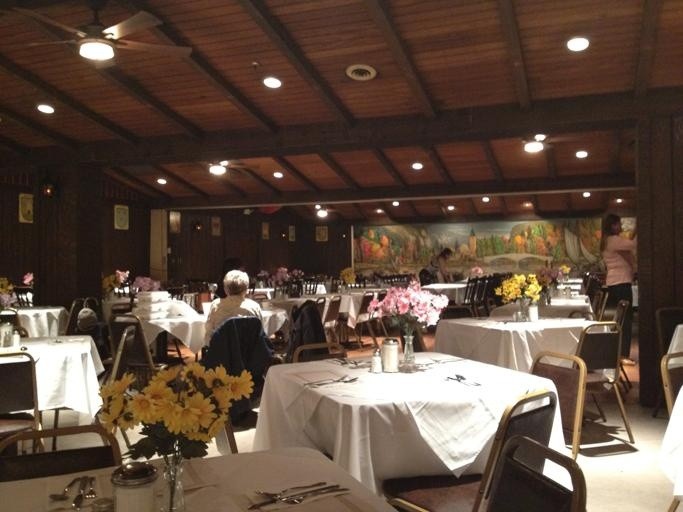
[318,376,360,388]
[85,476,96,503]
[304,373,349,386]
[281,487,351,504]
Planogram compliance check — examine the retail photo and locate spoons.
[456,373,481,386]
[253,480,326,501]
[50,478,80,500]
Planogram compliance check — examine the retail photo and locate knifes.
[446,376,468,387]
[72,476,88,509]
[249,482,339,511]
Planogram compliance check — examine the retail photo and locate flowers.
[130,277,160,294]
[495,272,543,304]
[0,277,18,311]
[367,281,449,336]
[98,360,255,462]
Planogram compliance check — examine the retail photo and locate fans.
[22,11,191,70]
[177,150,260,179]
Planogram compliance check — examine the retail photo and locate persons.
[419,248,454,286]
[204,270,263,352]
[601,214,638,366]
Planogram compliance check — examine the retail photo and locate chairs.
[572,321,635,445]
[388,390,558,512]
[592,288,609,321]
[450,273,512,318]
[167,287,186,300]
[315,297,326,319]
[0,352,45,454]
[304,277,318,295]
[83,296,99,308]
[527,351,587,460]
[323,295,342,343]
[64,298,84,336]
[111,304,132,314]
[330,278,341,292]
[195,291,211,313]
[660,352,683,418]
[96,324,136,390]
[585,278,603,306]
[485,435,587,512]
[375,274,408,286]
[579,272,592,295]
[109,314,168,372]
[359,289,390,342]
[354,277,366,288]
[0,425,123,482]
[0,309,23,336]
[13,326,29,338]
[338,290,379,352]
[273,281,288,299]
[251,294,268,300]
[613,300,630,328]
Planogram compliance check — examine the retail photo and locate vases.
[403,336,416,373]
[152,465,185,512]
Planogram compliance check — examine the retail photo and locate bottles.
[527,304,538,321]
[371,352,382,373]
[12,330,20,346]
[382,339,399,373]
[3,331,11,347]
